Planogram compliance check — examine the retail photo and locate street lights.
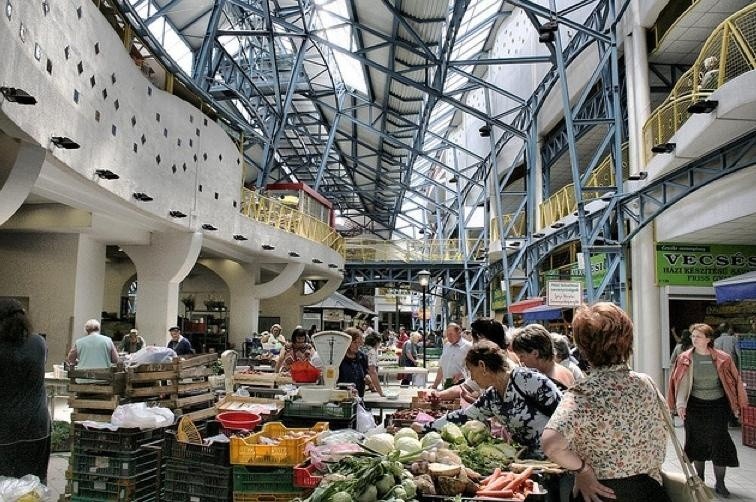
[416,268,429,368]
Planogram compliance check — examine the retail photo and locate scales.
[301,330,357,404]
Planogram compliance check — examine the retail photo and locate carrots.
[476,467,533,497]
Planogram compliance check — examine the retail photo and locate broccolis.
[441,419,487,445]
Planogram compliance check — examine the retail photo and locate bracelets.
[570,458,585,475]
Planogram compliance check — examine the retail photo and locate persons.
[158,325,194,400]
[433,322,472,391]
[662,318,744,429]
[336,323,437,400]
[675,323,747,497]
[540,299,677,502]
[66,319,119,389]
[407,340,563,462]
[0,298,52,489]
[503,324,584,388]
[119,328,145,354]
[260,323,319,372]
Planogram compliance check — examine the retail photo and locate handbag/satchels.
[660,469,718,502]
[404,358,415,366]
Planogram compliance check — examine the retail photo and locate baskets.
[233,490,312,502]
[233,465,303,492]
[735,339,756,448]
[65,425,173,501]
[293,464,323,489]
[229,421,330,467]
[279,403,356,431]
[163,424,231,501]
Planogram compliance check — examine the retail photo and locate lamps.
[473,95,720,254]
[536,18,558,44]
[448,175,457,183]
[419,228,424,234]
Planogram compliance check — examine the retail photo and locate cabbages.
[363,427,442,454]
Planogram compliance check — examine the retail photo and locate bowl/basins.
[299,383,333,404]
[215,411,262,431]
[290,368,322,383]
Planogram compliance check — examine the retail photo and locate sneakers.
[715,485,730,498]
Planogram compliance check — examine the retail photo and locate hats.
[0,298,26,317]
